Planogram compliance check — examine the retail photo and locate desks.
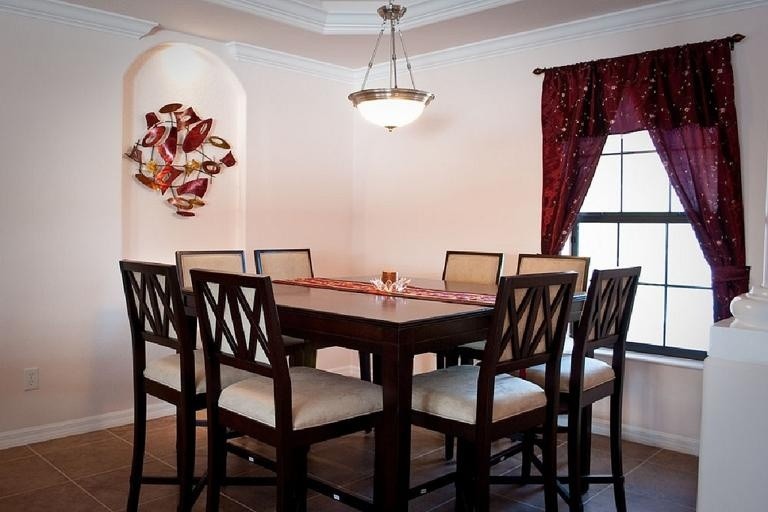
[173,276,591,512]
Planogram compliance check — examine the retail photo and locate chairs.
[436,251,503,369]
[457,254,591,366]
[119,259,261,512]
[254,248,315,281]
[410,271,580,511]
[176,250,247,292]
[510,266,642,512]
[189,267,382,512]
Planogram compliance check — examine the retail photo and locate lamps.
[345,0,436,135]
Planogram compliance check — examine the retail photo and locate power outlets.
[22,367,39,392]
